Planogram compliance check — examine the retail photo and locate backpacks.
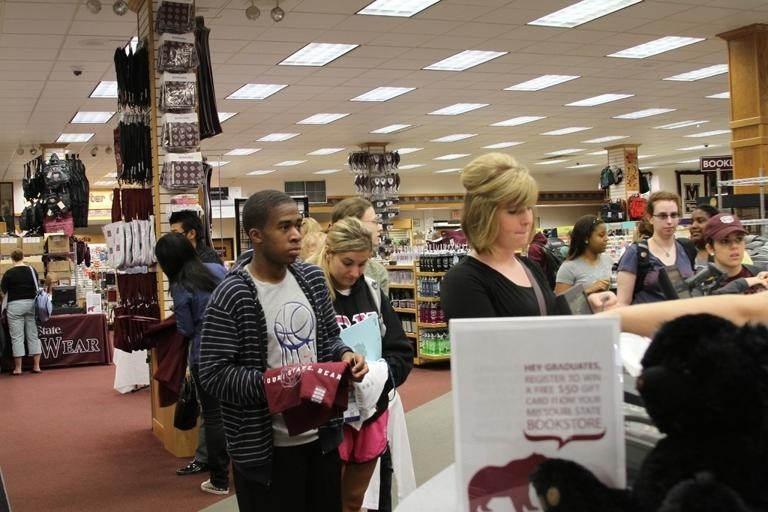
[627,193,648,218]
[34,287,54,323]
[534,236,572,291]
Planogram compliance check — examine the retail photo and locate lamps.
[245,6,259,20]
[17,147,115,158]
[270,6,284,22]
[113,0,129,16]
[87,0,101,13]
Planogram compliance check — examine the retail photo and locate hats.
[702,211,749,245]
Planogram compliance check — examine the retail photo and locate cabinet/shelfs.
[384,250,463,364]
[717,167,768,226]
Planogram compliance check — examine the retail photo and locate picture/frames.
[673,169,708,220]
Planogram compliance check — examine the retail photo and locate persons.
[315,217,416,510]
[156,233,231,496]
[167,211,223,477]
[530,191,768,512]
[199,187,368,512]
[37,271,61,320]
[441,153,615,321]
[1,249,43,375]
[329,194,391,511]
[297,215,327,264]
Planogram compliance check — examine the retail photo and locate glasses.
[177,231,187,236]
[590,216,601,231]
[360,216,380,226]
[652,212,679,219]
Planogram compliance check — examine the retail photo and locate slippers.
[9,370,22,376]
[30,368,42,374]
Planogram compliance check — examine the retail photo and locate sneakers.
[199,479,230,496]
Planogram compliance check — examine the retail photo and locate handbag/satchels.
[173,376,201,432]
[600,203,629,222]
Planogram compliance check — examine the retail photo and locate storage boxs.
[1,237,18,255]
[45,233,70,253]
[0,260,14,283]
[23,259,45,280]
[21,236,44,254]
[46,261,72,278]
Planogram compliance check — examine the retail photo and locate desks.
[1,313,113,374]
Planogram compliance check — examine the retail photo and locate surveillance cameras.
[105,146,113,155]
[576,162,580,166]
[704,143,708,147]
[90,149,99,157]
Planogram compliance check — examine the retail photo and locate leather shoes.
[176,459,212,476]
